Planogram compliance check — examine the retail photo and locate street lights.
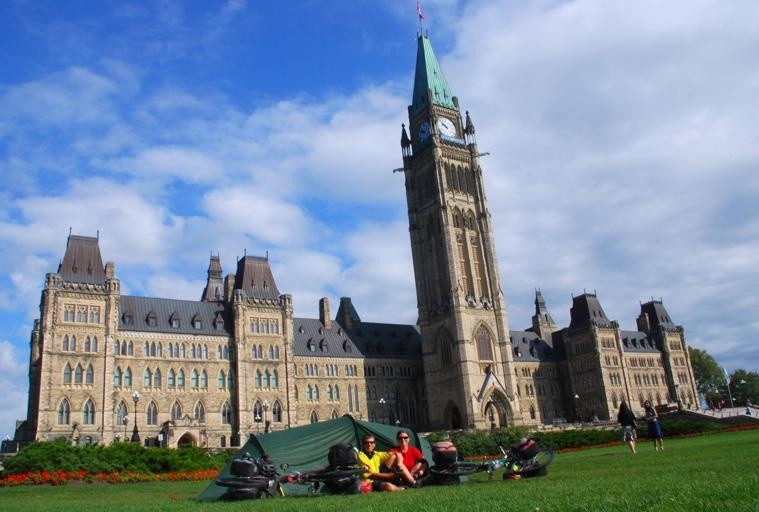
[715,380,747,407]
[573,394,582,424]
[379,398,401,427]
[115,390,140,442]
[254,400,269,435]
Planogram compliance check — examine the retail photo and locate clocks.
[418,122,431,143]
[437,116,457,138]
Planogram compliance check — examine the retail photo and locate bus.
[0,439,19,457]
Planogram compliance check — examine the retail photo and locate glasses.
[398,437,408,440]
[364,441,375,444]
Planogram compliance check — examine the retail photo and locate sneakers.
[412,474,433,488]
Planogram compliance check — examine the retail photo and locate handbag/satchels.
[633,420,637,427]
[328,443,358,466]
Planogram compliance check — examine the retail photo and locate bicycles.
[215,463,370,499]
[429,434,554,479]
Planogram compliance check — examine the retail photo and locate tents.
[192,413,470,503]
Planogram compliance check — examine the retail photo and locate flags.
[417,2,425,21]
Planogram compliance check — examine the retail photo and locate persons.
[642,400,664,452]
[356,434,424,493]
[617,402,638,455]
[384,430,433,486]
